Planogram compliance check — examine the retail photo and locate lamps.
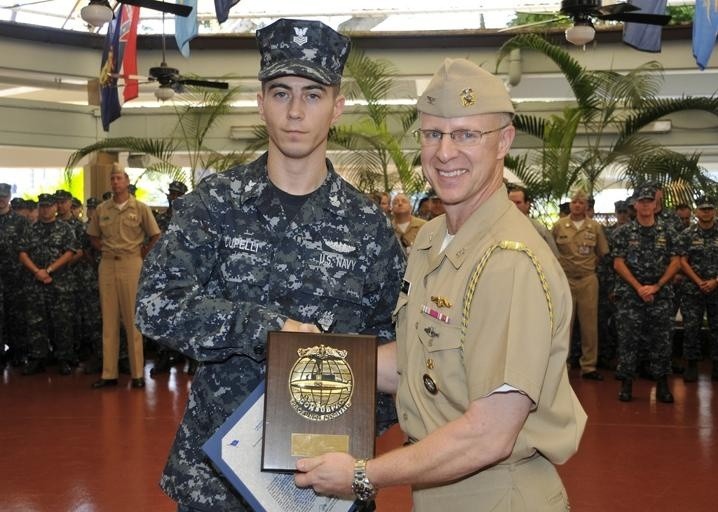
[561,17,599,48]
[154,85,177,103]
[80,1,117,32]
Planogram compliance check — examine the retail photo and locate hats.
[614,180,661,212]
[110,161,126,174]
[168,181,188,194]
[255,17,351,86]
[127,184,137,194]
[415,57,516,122]
[0,183,115,209]
[559,189,596,215]
[694,194,715,209]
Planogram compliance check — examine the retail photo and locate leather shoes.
[131,377,145,388]
[91,379,117,389]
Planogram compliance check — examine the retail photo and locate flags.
[98,0,240,131]
[690,0,717,70]
[620,0,667,53]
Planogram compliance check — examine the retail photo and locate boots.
[712,360,718,382]
[682,359,698,382]
[656,375,673,403]
[618,378,632,401]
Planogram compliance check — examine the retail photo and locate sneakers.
[581,371,604,381]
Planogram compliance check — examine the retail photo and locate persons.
[508,179,718,403]
[134,21,409,512]
[373,191,445,251]
[0,165,196,389]
[293,57,590,511]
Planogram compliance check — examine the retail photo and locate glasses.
[410,123,509,146]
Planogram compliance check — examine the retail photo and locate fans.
[77,0,197,24]
[106,1,233,95]
[498,0,679,36]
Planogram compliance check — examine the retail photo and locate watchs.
[352,457,378,503]
